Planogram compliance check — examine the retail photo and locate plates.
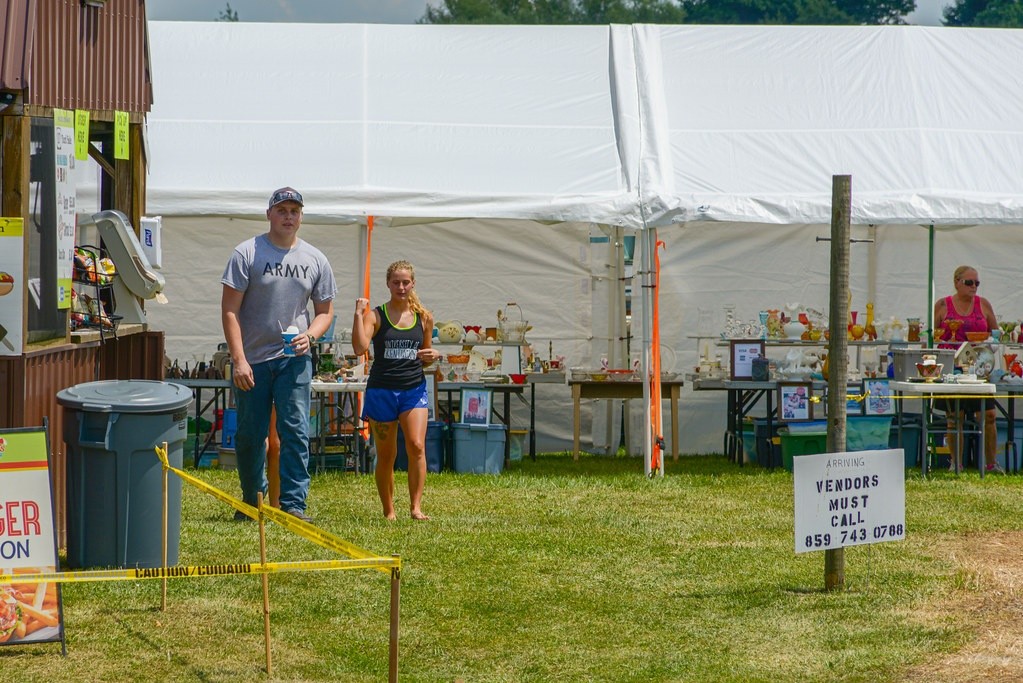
[459,350,488,374]
[479,376,504,383]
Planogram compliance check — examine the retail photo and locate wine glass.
[935,320,1023,344]
[439,355,453,384]
[786,348,829,373]
[447,354,470,383]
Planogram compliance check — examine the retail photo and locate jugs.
[434,321,462,343]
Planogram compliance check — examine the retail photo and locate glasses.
[957,279,980,287]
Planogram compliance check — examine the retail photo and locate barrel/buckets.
[498,302,528,343]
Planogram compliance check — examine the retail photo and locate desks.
[889,381,996,478]
[568,381,684,461]
[693,380,1023,467]
[910,377,1023,475]
[167,378,531,469]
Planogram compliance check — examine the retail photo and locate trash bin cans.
[56,379,193,571]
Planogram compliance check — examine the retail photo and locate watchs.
[304,332,316,348]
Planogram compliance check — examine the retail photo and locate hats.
[269,187,304,209]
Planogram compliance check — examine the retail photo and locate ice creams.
[283,325,299,334]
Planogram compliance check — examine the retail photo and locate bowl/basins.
[965,331,989,343]
[508,373,527,384]
[570,368,681,381]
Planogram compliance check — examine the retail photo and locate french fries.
[7,580,60,638]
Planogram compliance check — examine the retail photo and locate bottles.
[165,357,231,381]
[331,341,345,371]
[533,357,541,372]
[318,352,335,376]
[492,350,502,370]
[696,303,921,343]
[752,353,769,381]
[700,353,723,379]
[880,349,888,375]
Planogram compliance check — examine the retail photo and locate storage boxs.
[889,348,955,382]
[451,422,507,474]
[394,422,447,472]
[735,418,828,470]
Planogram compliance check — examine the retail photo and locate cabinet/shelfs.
[74,244,119,345]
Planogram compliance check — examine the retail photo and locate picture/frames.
[459,386,494,424]
[823,383,864,416]
[424,371,438,421]
[863,378,898,416]
[777,381,814,423]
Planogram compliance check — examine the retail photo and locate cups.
[549,360,560,370]
[463,325,482,343]
[465,369,481,383]
[486,327,496,341]
[324,315,337,342]
[280,332,299,357]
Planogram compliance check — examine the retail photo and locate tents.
[27,21,1023,478]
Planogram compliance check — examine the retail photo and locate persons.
[934,265,1005,475]
[351,260,440,520]
[220,186,339,524]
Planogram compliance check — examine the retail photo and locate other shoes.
[289,511,315,523]
[234,500,258,522]
[949,459,963,471]
[985,461,1005,476]
